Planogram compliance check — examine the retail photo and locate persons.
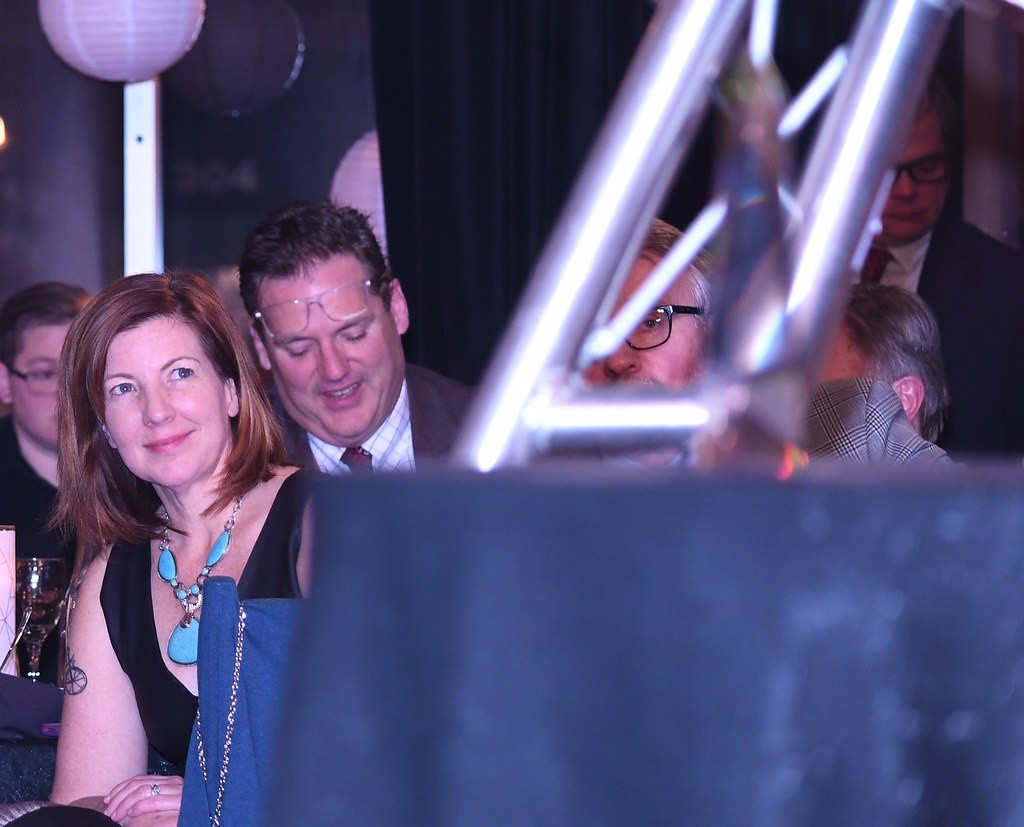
[813,279,1018,465]
[856,66,1024,457]
[1,284,94,687]
[576,218,961,470]
[238,198,478,474]
[37,271,316,827]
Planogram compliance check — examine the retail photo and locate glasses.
[2,359,58,395]
[893,152,954,185]
[623,303,706,350]
[250,265,391,337]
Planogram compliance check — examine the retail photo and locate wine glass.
[14,558,67,680]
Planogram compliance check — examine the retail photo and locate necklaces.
[157,492,243,666]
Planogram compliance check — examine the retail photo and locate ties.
[860,245,895,284]
[340,445,375,476]
